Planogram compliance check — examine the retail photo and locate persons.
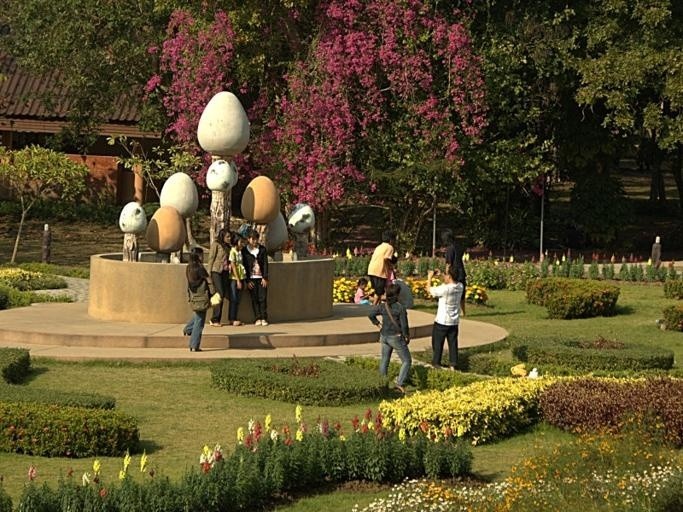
[426,263,464,372]
[352,229,399,308]
[369,283,413,395]
[228,232,247,327]
[182,247,215,352]
[440,225,466,318]
[207,228,231,328]
[241,229,269,327]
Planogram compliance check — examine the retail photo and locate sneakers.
[209,319,268,326]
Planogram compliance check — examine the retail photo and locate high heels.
[183,331,191,336]
[190,348,203,351]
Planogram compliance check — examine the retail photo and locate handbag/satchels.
[189,289,211,312]
